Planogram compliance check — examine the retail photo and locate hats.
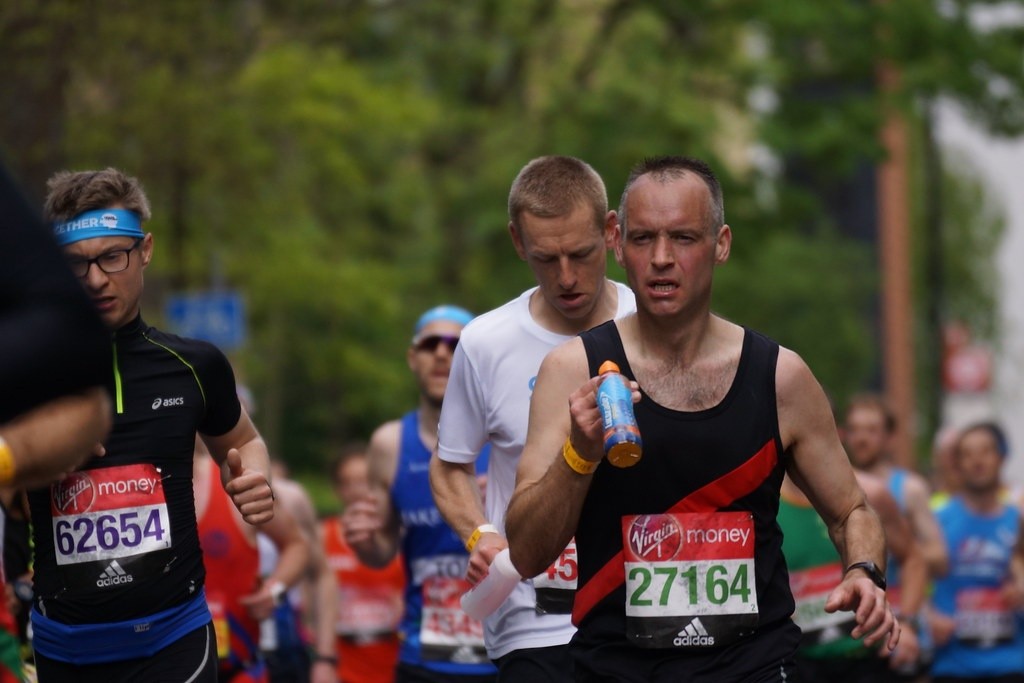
[414,305,475,328]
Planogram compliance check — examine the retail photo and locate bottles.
[595,360,643,467]
[460,547,522,621]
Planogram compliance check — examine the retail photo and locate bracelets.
[0,438,14,483]
[465,524,499,552]
[273,577,285,606]
[308,649,337,665]
[844,561,886,591]
[564,437,601,475]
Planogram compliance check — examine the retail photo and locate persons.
[506,157,901,683]
[0,166,336,683]
[427,158,636,683]
[916,425,1024,683]
[776,390,949,683]
[306,446,407,683]
[340,304,500,683]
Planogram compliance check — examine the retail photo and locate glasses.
[67,238,143,279]
[412,332,459,352]
